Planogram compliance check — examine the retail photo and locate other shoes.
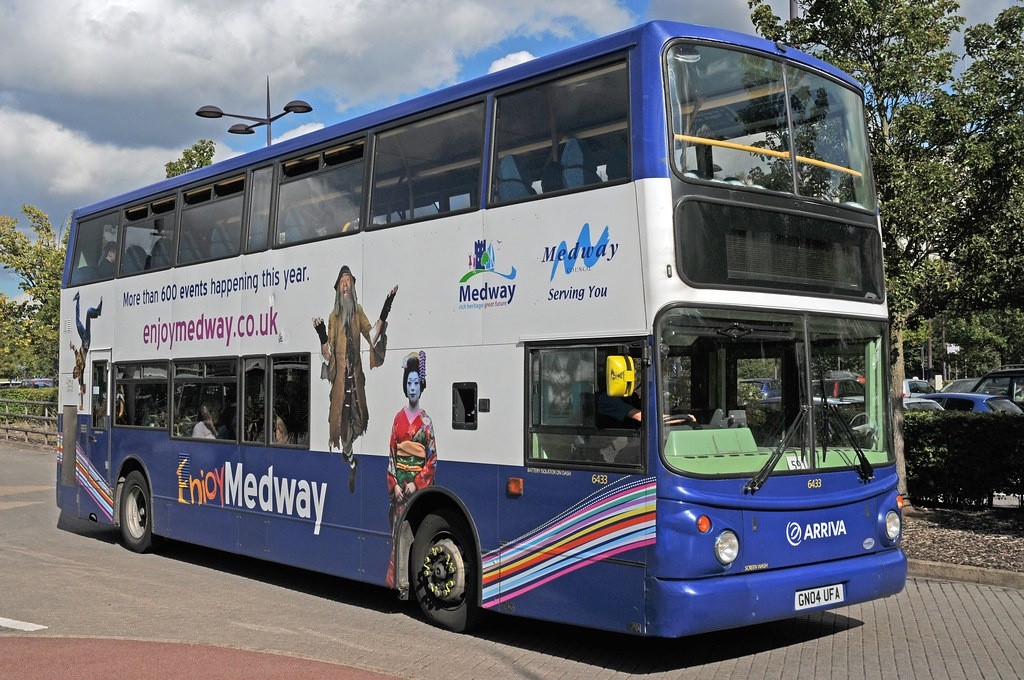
[73,291,80,301]
[343,452,360,492]
[97,300,102,316]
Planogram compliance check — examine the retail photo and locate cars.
[16,380,50,388]
[724,362,1024,447]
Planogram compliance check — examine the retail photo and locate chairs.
[70,137,629,291]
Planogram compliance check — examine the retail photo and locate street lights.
[195,73,313,148]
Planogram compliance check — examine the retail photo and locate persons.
[99,360,310,451]
[97,241,118,277]
[69,291,103,409]
[596,392,696,427]
[387,350,437,587]
[313,265,399,493]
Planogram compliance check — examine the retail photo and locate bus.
[59,20,909,642]
